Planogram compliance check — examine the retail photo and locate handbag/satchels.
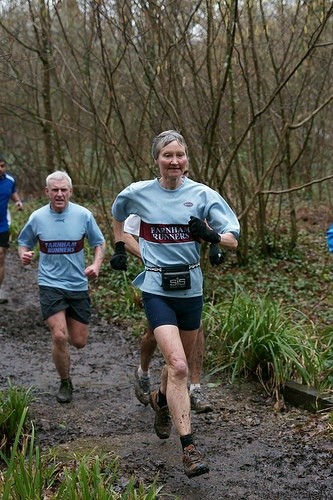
[161,265,191,292]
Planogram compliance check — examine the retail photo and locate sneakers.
[182,445,209,478]
[151,391,172,439]
[57,378,73,403]
[134,366,152,405]
[189,389,214,413]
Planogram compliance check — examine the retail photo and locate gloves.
[209,244,223,267]
[110,241,128,271]
[188,215,219,242]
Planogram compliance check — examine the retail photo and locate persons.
[17,171,106,402]
[110,129,240,479]
[0,156,24,304]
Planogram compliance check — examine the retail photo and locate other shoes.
[0,299,8,304]
